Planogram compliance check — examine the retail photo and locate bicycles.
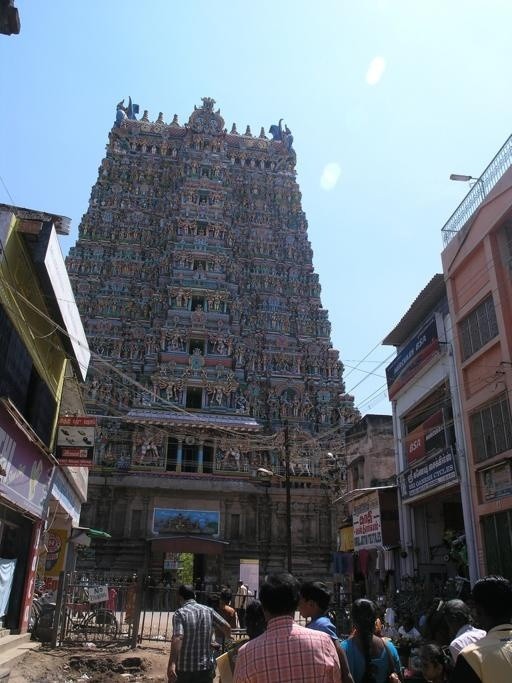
[30,588,118,648]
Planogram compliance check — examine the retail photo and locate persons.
[483,472,497,490]
[136,427,161,467]
[104,586,117,615]
[220,434,241,466]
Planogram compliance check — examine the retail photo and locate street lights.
[257,467,292,576]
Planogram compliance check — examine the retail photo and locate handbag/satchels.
[388,670,402,683]
